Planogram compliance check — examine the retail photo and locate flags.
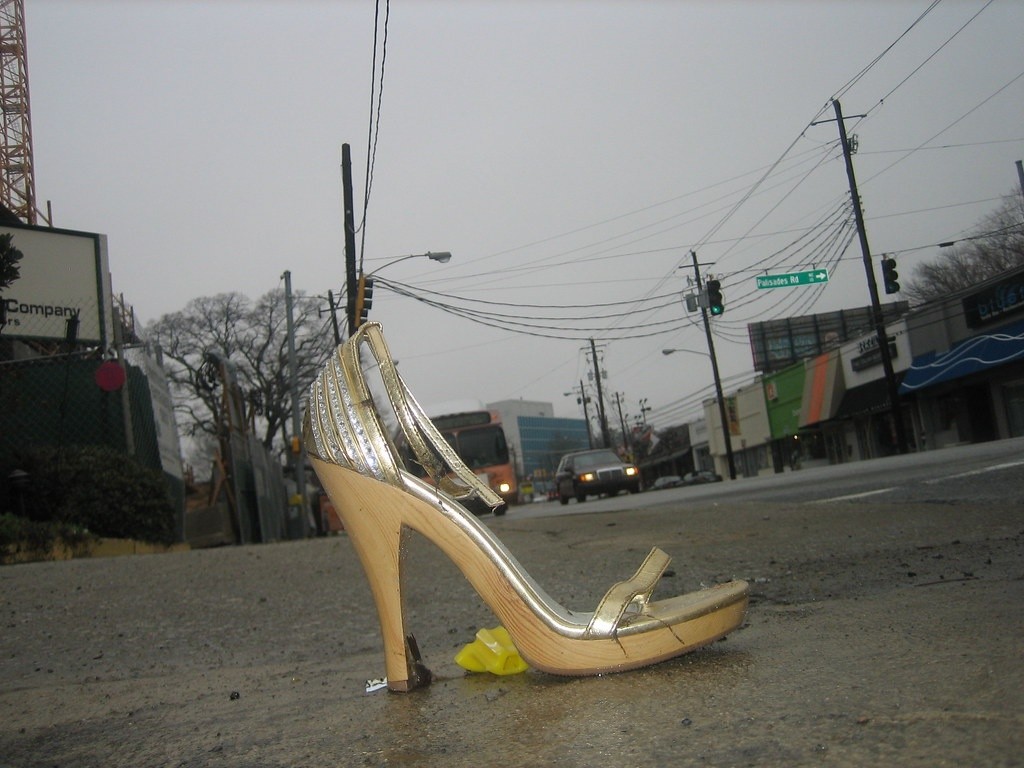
[645,435,661,456]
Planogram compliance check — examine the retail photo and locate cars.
[647,476,684,490]
[682,471,723,485]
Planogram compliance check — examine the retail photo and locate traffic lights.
[355,307,369,330]
[707,280,725,316]
[881,257,900,294]
[358,276,374,311]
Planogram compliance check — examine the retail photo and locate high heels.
[302,322,751,693]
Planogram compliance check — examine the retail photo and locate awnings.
[898,319,1024,394]
[798,350,846,427]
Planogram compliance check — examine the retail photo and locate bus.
[389,409,516,511]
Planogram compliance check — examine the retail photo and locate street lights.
[346,251,454,336]
[562,391,595,451]
[662,347,736,480]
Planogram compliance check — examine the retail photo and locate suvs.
[554,448,640,504]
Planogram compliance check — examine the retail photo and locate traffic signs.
[756,268,829,289]
